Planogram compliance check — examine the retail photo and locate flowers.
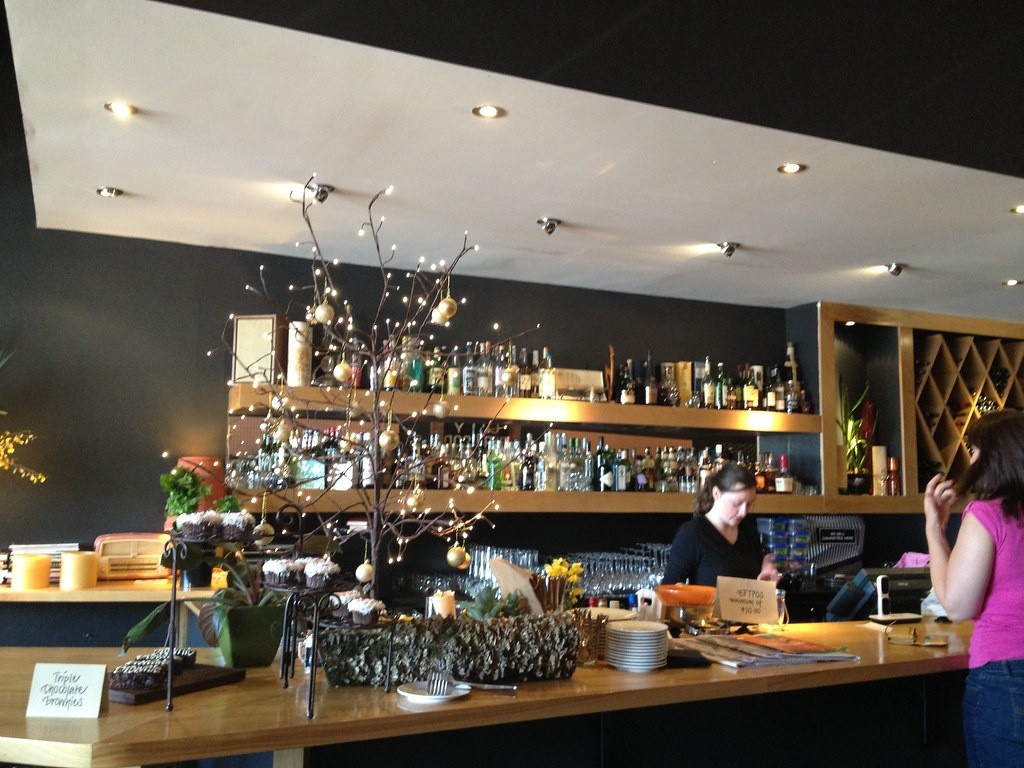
[546,559,584,603]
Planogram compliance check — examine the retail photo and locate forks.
[428,678,516,695]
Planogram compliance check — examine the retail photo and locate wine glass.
[452,542,673,598]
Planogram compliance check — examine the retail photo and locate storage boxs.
[677,361,706,404]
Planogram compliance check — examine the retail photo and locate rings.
[776,573,782,578]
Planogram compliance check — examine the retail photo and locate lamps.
[716,241,740,258]
[307,183,335,203]
[884,262,905,276]
[536,217,561,234]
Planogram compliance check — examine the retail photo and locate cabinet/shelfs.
[783,300,1024,497]
[223,383,825,516]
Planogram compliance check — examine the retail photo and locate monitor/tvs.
[826,566,933,624]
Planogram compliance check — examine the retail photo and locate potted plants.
[318,587,578,686]
[836,380,880,495]
[118,460,288,670]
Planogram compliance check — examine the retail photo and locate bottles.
[348,334,812,413]
[914,356,1010,493]
[229,427,795,494]
[882,456,900,496]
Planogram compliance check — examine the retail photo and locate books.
[671,630,858,669]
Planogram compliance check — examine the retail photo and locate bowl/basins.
[654,582,718,629]
[758,516,810,571]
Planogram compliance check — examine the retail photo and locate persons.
[923,408,1024,768]
[659,464,783,586]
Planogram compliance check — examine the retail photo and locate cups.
[776,589,785,626]
[396,572,462,597]
[60,549,98,593]
[574,632,598,667]
[10,554,51,590]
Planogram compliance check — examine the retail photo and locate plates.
[396,681,472,704]
[562,606,670,672]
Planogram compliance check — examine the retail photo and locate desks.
[0,576,220,648]
